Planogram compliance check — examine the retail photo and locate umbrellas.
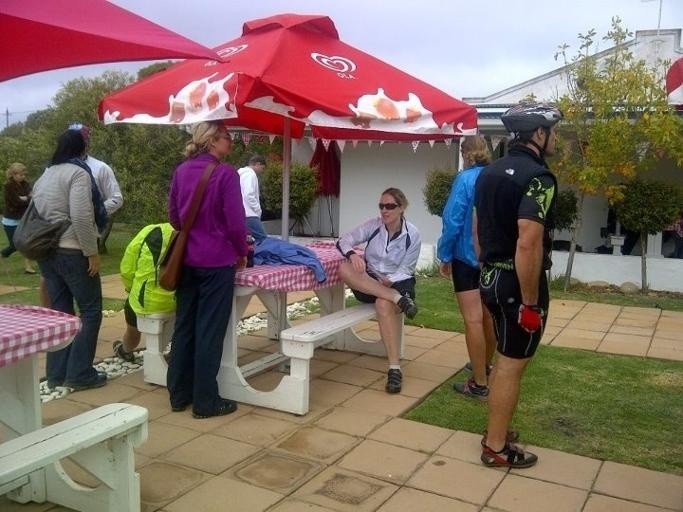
[309,139,326,237]
[324,141,340,237]
[98,15,482,245]
[0,0,229,84]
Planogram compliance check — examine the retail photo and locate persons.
[337,187,422,393]
[0,163,38,274]
[39,124,123,308]
[113,221,179,363]
[472,103,564,469]
[32,130,107,390]
[437,136,498,397]
[167,121,251,419]
[236,155,267,241]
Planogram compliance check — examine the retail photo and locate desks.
[0,304,83,433]
[221,242,365,377]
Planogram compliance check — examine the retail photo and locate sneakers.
[112,340,135,362]
[481,429,520,447]
[170,402,185,411]
[192,396,237,419]
[453,376,489,401]
[397,289,417,320]
[465,363,493,375]
[386,368,402,392]
[70,374,106,389]
[481,442,538,467]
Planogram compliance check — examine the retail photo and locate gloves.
[518,304,542,334]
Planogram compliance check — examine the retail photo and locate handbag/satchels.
[158,161,218,291]
[12,200,72,262]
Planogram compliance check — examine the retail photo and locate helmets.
[501,101,565,133]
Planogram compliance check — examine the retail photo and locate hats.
[68,124,90,140]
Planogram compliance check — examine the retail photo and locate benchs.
[0,402,148,512]
[280,293,405,416]
[136,313,177,386]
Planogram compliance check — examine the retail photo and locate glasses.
[379,203,398,210]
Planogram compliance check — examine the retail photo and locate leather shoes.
[25,270,37,274]
